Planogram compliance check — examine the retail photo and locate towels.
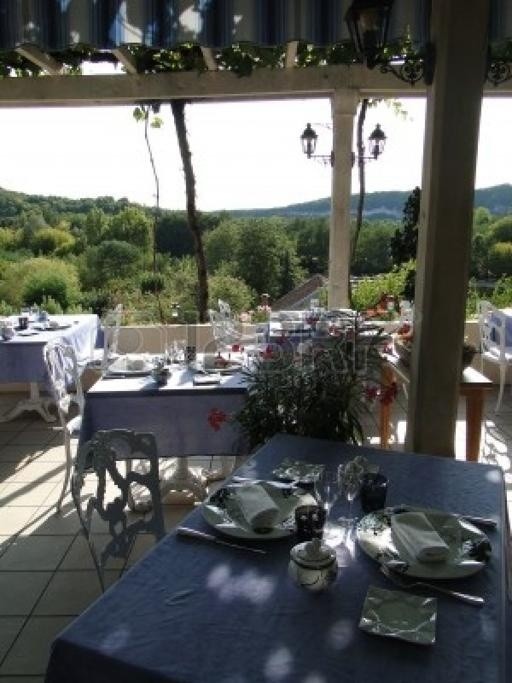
[232,482,281,527]
[390,510,451,563]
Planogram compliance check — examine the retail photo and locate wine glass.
[315,453,372,528]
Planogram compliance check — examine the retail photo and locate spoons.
[374,556,485,608]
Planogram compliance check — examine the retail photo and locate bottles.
[289,539,339,598]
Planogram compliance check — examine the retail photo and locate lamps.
[351,120,387,168]
[299,119,336,168]
[342,0,437,87]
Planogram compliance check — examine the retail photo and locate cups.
[358,472,389,511]
[293,504,327,542]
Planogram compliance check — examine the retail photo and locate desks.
[379,351,495,462]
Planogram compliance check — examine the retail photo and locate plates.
[356,502,492,579]
[358,585,437,649]
[202,475,318,539]
[0,302,80,341]
[274,453,325,486]
[273,296,415,332]
[103,341,271,384]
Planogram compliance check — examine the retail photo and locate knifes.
[175,524,269,556]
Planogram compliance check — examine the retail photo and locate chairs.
[38,337,138,510]
[476,300,512,411]
[86,303,123,377]
[69,424,167,596]
[208,298,414,382]
